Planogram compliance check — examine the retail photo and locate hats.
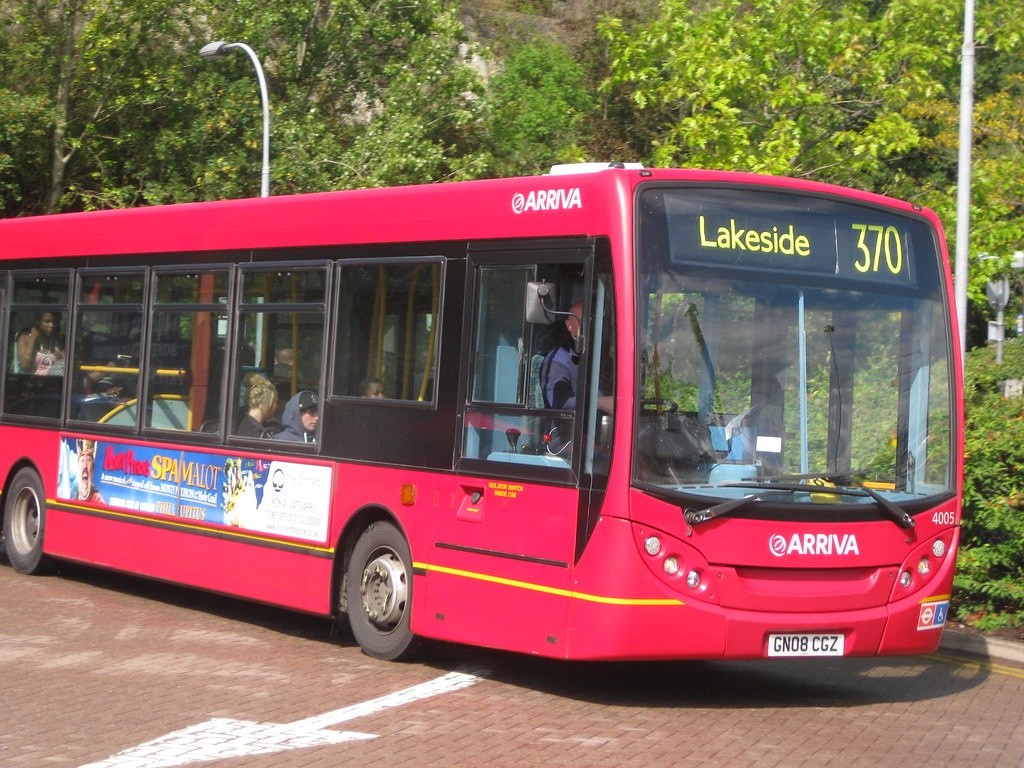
[299,392,319,411]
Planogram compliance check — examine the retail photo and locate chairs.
[1,266,788,487]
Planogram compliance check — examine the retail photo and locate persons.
[17,312,64,375]
[358,374,385,398]
[538,302,614,467]
[240,334,255,366]
[274,349,315,384]
[236,372,278,438]
[80,360,123,396]
[271,389,318,443]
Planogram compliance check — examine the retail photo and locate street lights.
[199,40,270,198]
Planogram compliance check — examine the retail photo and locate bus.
[0,158,967,663]
